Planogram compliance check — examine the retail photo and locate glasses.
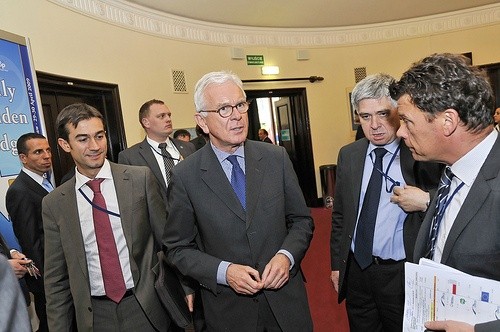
[198,101,251,117]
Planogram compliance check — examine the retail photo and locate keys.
[20,258,42,280]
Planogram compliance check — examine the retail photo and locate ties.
[84,178,126,304]
[226,155,247,213]
[421,168,454,258]
[353,147,388,270]
[42,178,54,192]
[159,142,175,185]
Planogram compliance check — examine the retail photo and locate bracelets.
[423,192,430,212]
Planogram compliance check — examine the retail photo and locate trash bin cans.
[319,164,337,209]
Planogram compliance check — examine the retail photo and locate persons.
[59,154,107,186]
[162,71,317,332]
[43,104,195,332]
[390,53,500,332]
[6,132,56,332]
[117,99,197,194]
[173,123,210,150]
[328,72,446,332]
[494,107,500,135]
[259,129,273,145]
[354,110,366,141]
[0,231,32,332]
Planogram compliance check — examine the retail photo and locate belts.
[374,257,400,265]
[91,290,134,301]
